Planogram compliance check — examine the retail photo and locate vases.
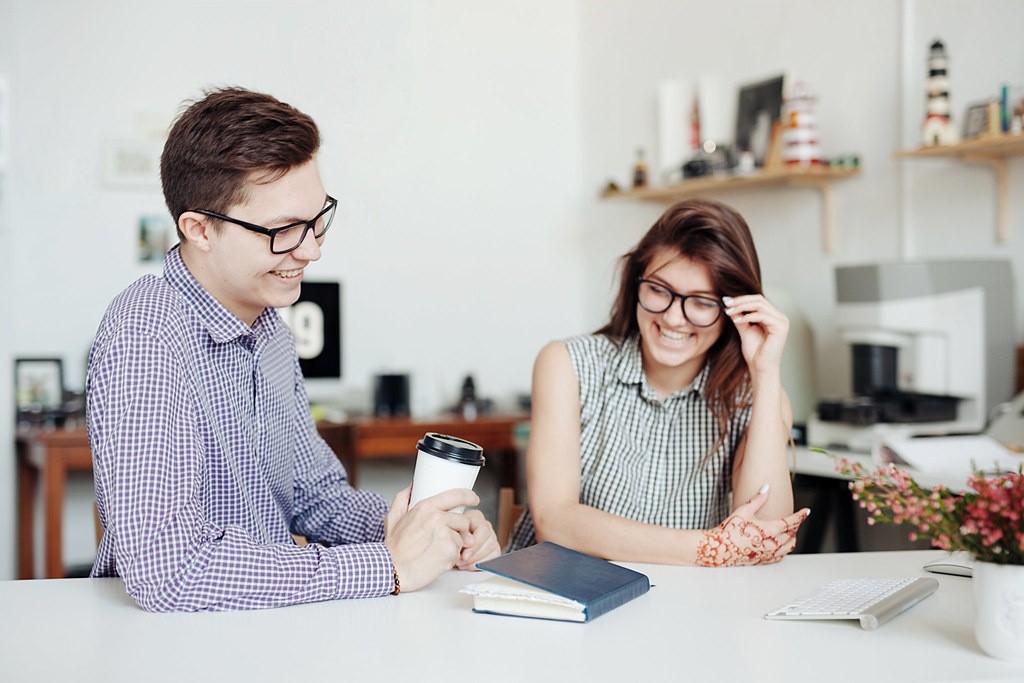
[972,561,1024,661]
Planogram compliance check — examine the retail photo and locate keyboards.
[764,577,939,631]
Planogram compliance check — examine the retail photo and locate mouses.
[922,558,975,577]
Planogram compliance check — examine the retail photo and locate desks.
[0,411,1024,682]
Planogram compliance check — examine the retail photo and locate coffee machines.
[799,260,1019,457]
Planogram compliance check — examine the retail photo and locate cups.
[406,432,486,513]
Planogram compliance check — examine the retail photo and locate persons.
[529,197,809,566]
[86,88,501,613]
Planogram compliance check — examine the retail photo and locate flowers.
[794,430,1024,566]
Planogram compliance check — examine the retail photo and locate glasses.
[194,194,338,254]
[635,275,727,327]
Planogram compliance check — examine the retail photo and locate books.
[461,541,651,624]
